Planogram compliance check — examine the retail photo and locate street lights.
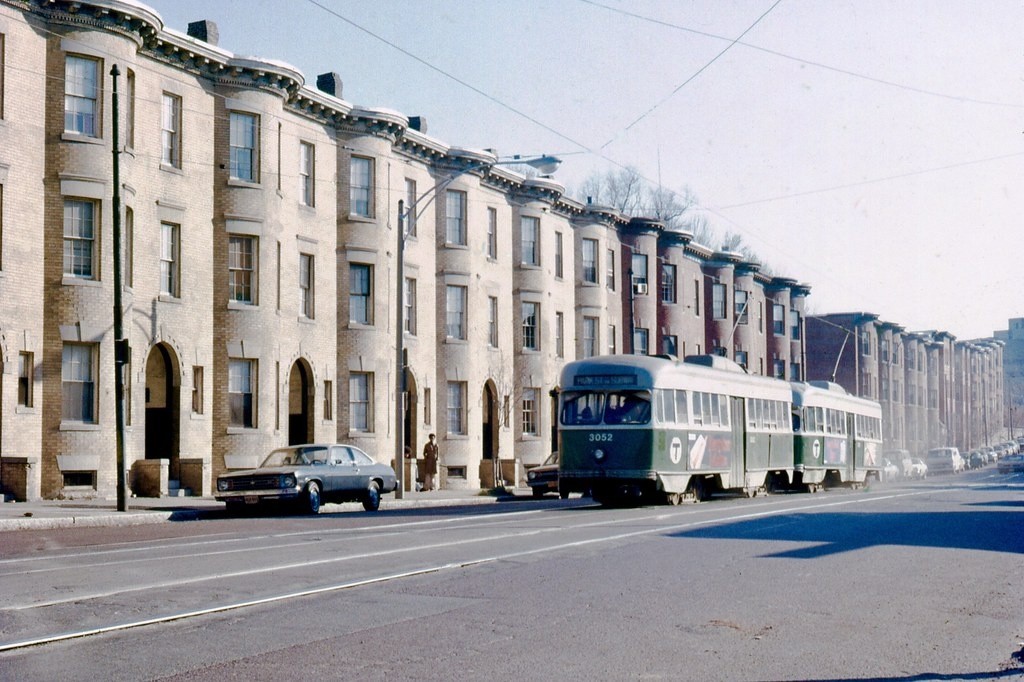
[1009,376,1014,440]
[110,63,125,513]
[627,269,634,354]
[395,157,561,499]
[887,332,924,449]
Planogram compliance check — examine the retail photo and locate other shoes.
[431,487,434,490]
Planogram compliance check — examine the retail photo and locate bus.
[557,353,883,507]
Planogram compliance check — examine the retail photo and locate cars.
[997,454,1024,474]
[959,436,1024,471]
[883,459,898,481]
[527,450,562,498]
[216,443,400,517]
[911,458,928,479]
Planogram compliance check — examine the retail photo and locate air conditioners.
[634,284,647,294]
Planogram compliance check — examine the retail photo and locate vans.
[926,448,961,474]
[884,449,915,481]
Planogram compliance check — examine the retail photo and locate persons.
[403,446,427,492]
[423,433,439,493]
